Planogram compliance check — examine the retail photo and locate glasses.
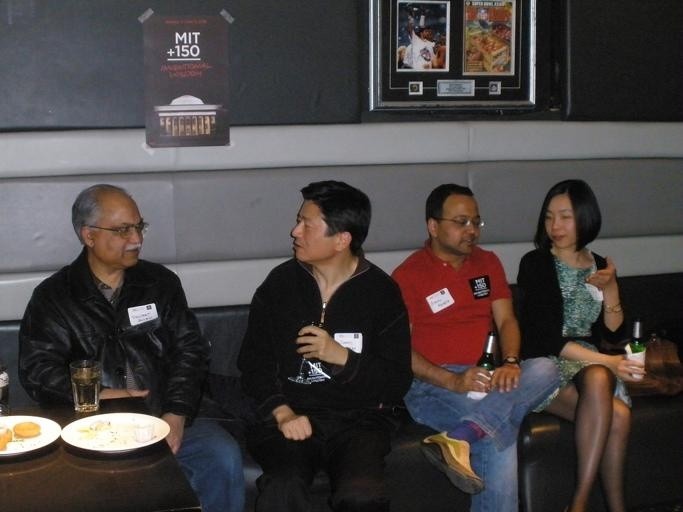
[437,217,486,231]
[88,223,150,239]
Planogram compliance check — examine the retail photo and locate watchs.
[499,356,521,366]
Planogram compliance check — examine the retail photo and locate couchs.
[0,273,682,512]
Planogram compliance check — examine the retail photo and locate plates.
[61,412,170,454]
[0,415,62,457]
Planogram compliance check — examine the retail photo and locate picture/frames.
[368,0,537,110]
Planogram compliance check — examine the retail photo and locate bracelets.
[602,302,623,313]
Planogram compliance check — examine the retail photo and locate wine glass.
[288,321,328,385]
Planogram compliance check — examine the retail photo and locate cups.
[70,359,102,413]
[0,367,9,415]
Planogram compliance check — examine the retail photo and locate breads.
[13,422,41,436]
[6,429,12,442]
[0,433,7,451]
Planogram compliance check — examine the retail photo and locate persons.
[390,183,561,512]
[398,16,445,70]
[517,179,648,512]
[236,180,413,511]
[18,184,246,512]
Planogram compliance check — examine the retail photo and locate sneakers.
[420,430,486,495]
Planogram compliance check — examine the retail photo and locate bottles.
[650,333,657,343]
[467,331,496,400]
[626,319,646,380]
